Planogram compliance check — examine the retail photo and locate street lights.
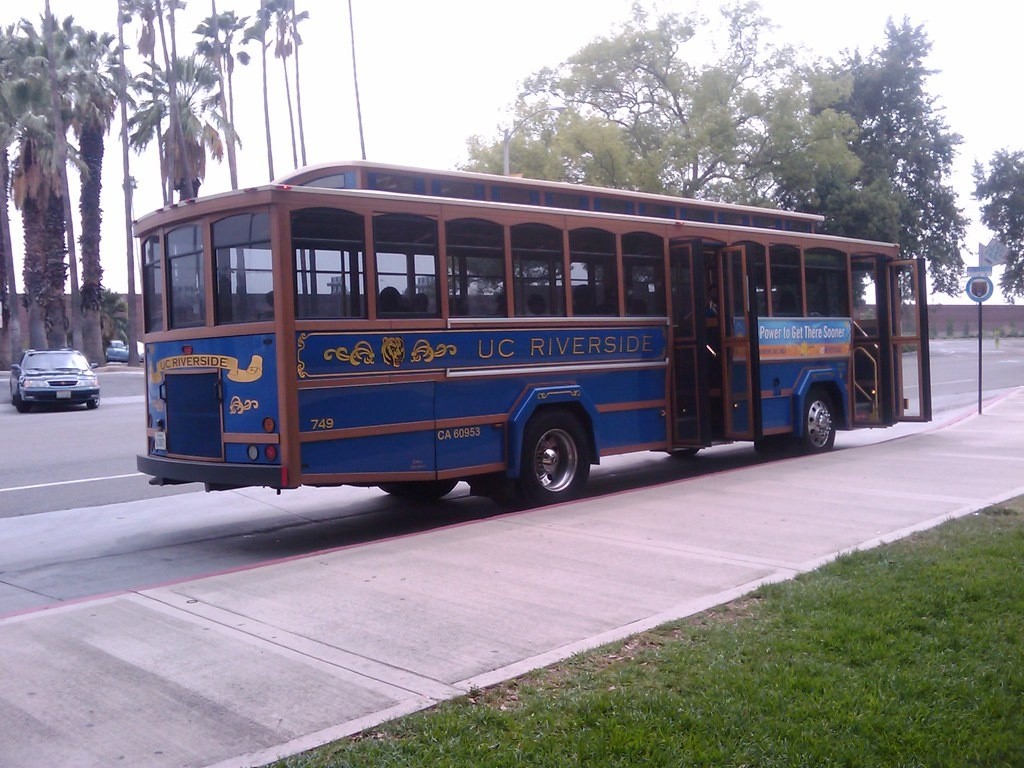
[503,106,566,177]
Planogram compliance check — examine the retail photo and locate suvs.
[9,348,101,413]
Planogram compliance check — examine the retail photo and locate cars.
[105,340,145,363]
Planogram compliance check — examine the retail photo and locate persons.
[573,284,618,313]
[705,285,732,425]
[529,294,546,315]
[380,287,430,313]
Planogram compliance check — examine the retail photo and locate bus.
[131,160,932,504]
